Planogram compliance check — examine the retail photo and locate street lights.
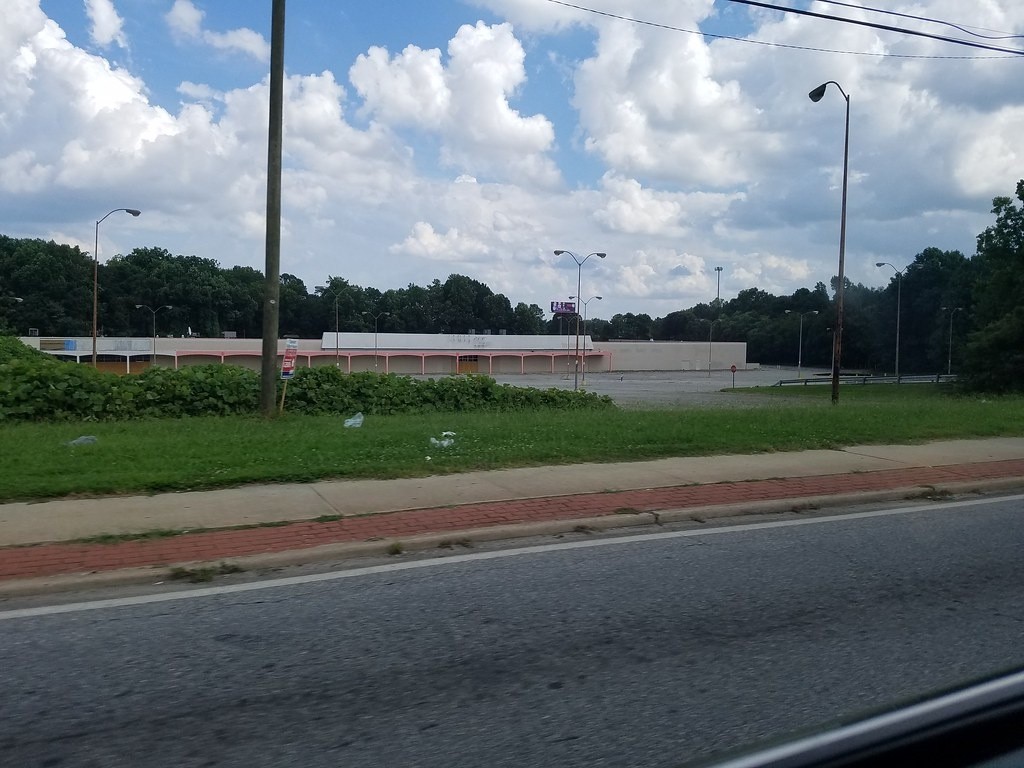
[361,311,390,374]
[553,250,607,391]
[784,310,819,379]
[826,326,844,376]
[700,318,722,377]
[92,208,141,367]
[940,306,963,375]
[136,304,173,364]
[875,263,925,377]
[714,266,723,298]
[808,81,850,406]
[315,285,359,370]
